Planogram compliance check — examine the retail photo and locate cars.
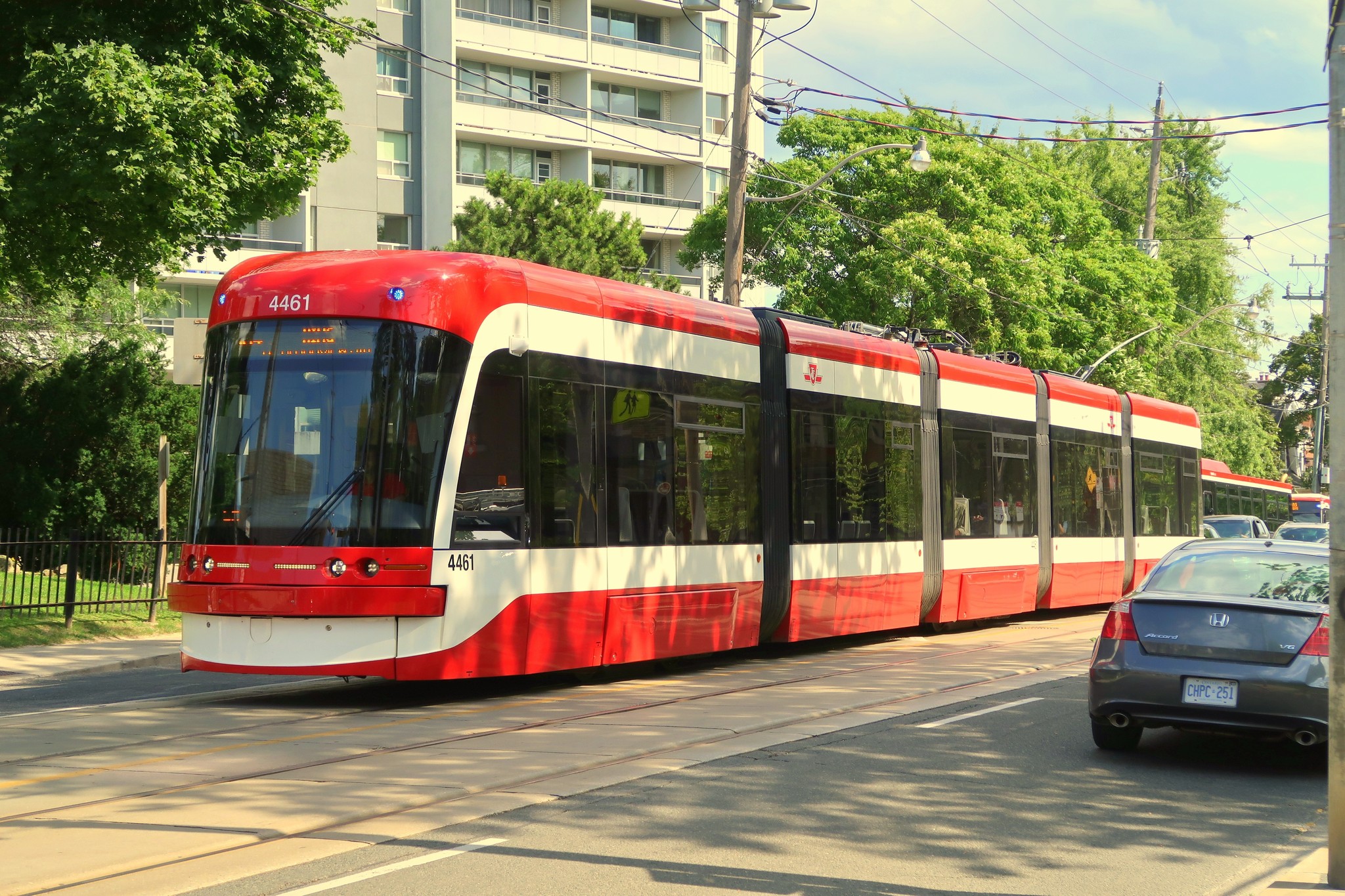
[1203,524,1221,540]
[1088,540,1331,753]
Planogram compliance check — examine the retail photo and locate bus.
[166,249,1202,687]
[1199,458,1331,539]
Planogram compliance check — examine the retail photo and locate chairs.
[1136,505,1189,536]
[803,520,871,543]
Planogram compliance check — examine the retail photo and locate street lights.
[722,135,932,307]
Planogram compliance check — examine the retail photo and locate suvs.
[1271,521,1329,544]
[1203,514,1270,540]
